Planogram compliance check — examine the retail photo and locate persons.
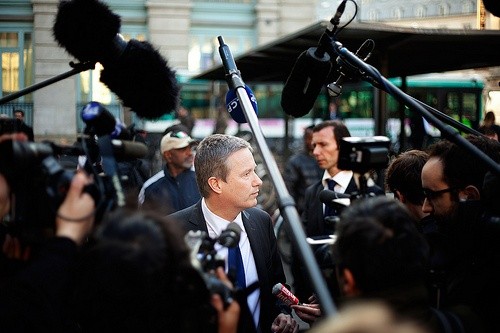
[271,134,500,333]
[159,134,301,333]
[136,129,202,220]
[285,124,327,213]
[0,109,240,332]
[477,111,500,141]
[291,123,387,308]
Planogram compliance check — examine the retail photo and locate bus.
[132,72,487,153]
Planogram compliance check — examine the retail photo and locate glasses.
[422,186,456,200]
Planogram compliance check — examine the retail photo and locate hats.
[160,130,201,154]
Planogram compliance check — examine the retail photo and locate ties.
[325,179,338,217]
[228,244,246,291]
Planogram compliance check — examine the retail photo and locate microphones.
[218,36,258,123]
[281,2,346,118]
[326,39,370,98]
[52,0,182,120]
[272,283,302,306]
